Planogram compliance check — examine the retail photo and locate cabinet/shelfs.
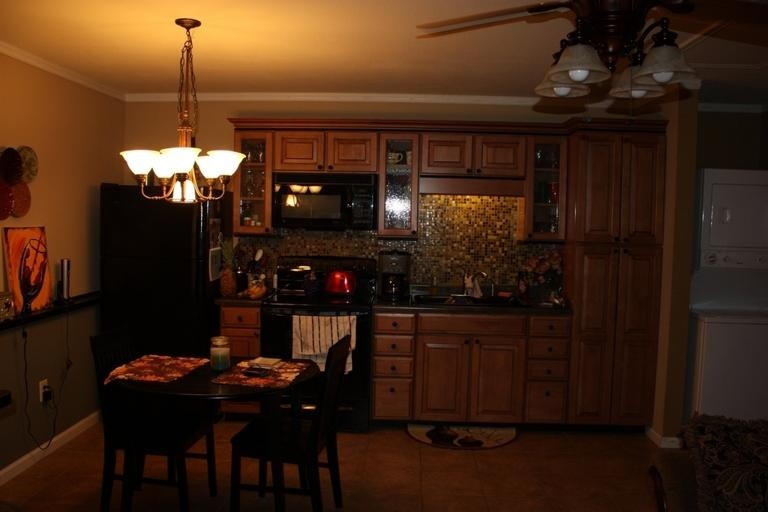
[371,311,417,424]
[412,310,529,426]
[220,303,261,358]
[270,118,379,179]
[564,116,670,247]
[227,118,274,236]
[378,118,420,239]
[524,118,568,244]
[525,311,571,424]
[416,120,527,180]
[561,242,663,428]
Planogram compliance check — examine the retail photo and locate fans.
[416,0,696,30]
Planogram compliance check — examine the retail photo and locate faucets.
[472,271,488,284]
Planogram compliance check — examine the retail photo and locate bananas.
[237,280,267,300]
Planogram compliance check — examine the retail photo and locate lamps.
[119,17,248,203]
[533,13,704,98]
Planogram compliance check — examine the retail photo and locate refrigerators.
[96,182,234,422]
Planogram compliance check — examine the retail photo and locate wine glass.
[20,238,48,315]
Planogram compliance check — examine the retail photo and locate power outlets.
[39,378,49,402]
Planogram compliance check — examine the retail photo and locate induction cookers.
[261,290,370,308]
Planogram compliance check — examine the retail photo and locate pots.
[324,267,357,295]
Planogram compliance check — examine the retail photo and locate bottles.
[240,144,265,227]
[489,282,495,296]
[60,258,71,300]
[431,276,438,296]
[57,281,63,301]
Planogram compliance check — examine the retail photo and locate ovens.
[261,308,372,432]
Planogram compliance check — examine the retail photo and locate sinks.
[411,294,469,306]
[470,296,523,307]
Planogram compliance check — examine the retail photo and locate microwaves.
[272,172,379,231]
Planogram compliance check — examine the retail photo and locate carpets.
[407,422,516,450]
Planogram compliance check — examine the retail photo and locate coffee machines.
[377,249,413,303]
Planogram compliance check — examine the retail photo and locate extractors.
[418,176,525,198]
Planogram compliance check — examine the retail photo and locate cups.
[0,292,15,321]
[387,151,404,164]
[534,150,559,234]
[405,151,412,165]
[236,267,264,291]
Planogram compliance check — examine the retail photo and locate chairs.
[85,335,219,511]
[230,333,351,511]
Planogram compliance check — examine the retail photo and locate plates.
[242,368,273,377]
[0,145,40,220]
[497,292,512,298]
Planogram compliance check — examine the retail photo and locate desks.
[113,360,319,497]
